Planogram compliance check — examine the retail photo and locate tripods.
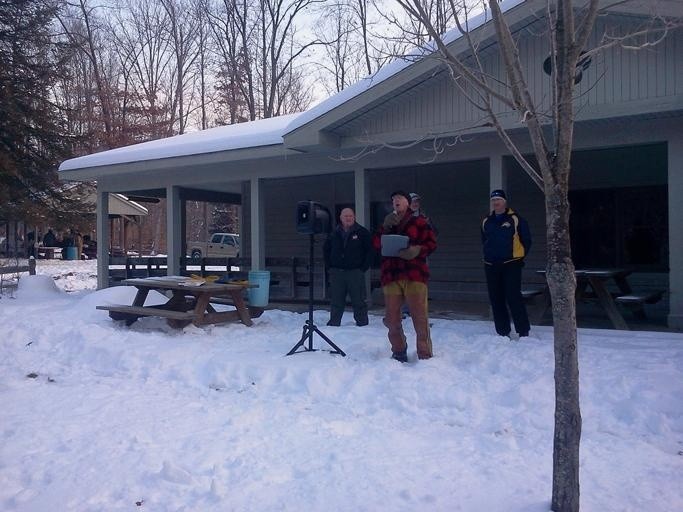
[286,236,346,357]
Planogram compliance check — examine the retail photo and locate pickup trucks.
[185,233,239,258]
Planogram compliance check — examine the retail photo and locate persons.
[372,190,438,362]
[323,208,371,326]
[29,229,98,262]
[480,189,532,336]
[410,190,440,240]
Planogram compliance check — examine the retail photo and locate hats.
[490,189,508,202]
[390,190,412,206]
[410,192,420,201]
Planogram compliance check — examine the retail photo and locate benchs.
[38,246,64,259]
[96,305,197,322]
[616,290,665,305]
[185,296,278,311]
[520,290,547,301]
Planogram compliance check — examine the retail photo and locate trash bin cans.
[66,246,78,260]
[247,270,271,308]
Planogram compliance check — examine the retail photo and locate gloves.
[399,245,422,260]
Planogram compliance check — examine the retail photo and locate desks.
[535,269,632,330]
[121,276,259,329]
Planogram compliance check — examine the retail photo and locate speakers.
[296,201,332,234]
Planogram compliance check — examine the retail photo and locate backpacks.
[482,222,513,261]
